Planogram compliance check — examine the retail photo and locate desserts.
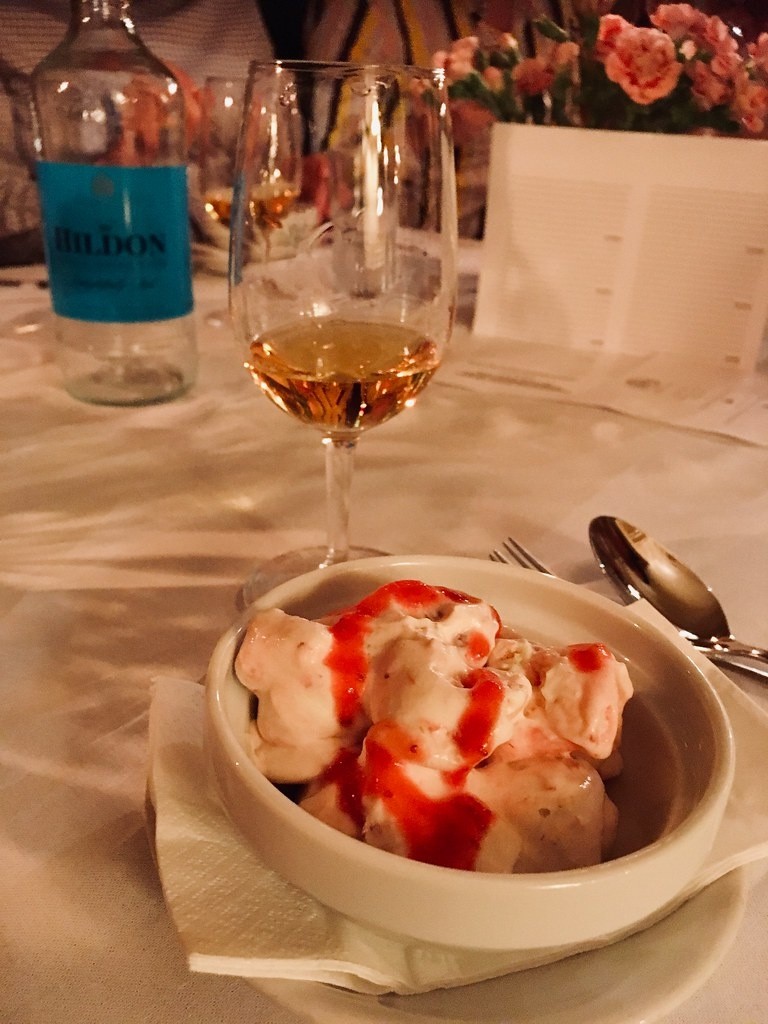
[233,578,636,872]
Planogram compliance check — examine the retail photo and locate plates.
[143,774,746,1024]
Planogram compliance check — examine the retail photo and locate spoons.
[587,515,768,665]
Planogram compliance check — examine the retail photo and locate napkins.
[148,597,768,997]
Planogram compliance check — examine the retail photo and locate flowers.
[431,3,768,137]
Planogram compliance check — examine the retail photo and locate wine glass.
[227,58,459,608]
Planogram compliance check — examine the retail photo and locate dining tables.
[0,224,768,1024]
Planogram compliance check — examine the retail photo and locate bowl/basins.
[205,556,738,951]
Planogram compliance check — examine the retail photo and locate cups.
[201,77,301,236]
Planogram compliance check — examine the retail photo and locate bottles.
[24,0,196,407]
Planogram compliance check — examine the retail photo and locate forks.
[490,536,768,679]
